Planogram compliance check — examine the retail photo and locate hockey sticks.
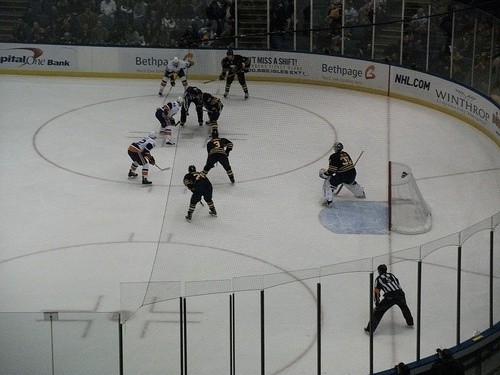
[163,74,178,103]
[145,155,171,171]
[173,121,180,126]
[200,201,204,206]
[203,69,251,84]
[332,150,364,196]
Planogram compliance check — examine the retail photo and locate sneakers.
[208,210,216,217]
[159,89,162,96]
[185,214,191,223]
[231,180,234,185]
[159,120,211,146]
[245,92,248,99]
[141,177,152,185]
[224,91,228,98]
[128,171,138,177]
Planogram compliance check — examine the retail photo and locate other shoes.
[364,327,369,335]
[408,323,412,327]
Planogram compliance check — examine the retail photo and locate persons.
[11,0,237,50]
[159,56,194,97]
[217,49,252,101]
[127,131,157,187]
[269,0,390,61]
[180,87,204,126]
[363,264,414,334]
[386,0,500,89]
[432,348,459,375]
[155,97,184,146]
[183,164,218,221]
[395,362,410,375]
[195,89,224,138]
[318,142,367,207]
[202,130,236,185]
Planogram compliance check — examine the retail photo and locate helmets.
[227,50,233,56]
[211,130,218,138]
[174,57,179,63]
[149,131,156,139]
[333,143,343,152]
[188,165,196,172]
[378,264,387,273]
[177,87,213,106]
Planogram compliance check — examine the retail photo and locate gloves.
[171,78,175,86]
[196,107,201,112]
[170,117,175,124]
[219,72,225,80]
[243,67,249,74]
[164,109,168,115]
[148,156,156,166]
